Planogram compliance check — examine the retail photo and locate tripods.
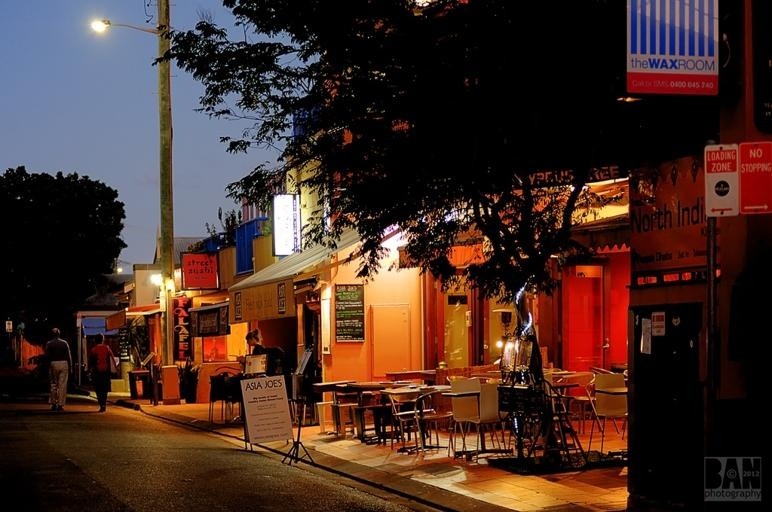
[281,381,316,467]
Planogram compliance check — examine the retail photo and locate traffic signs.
[702,139,772,511]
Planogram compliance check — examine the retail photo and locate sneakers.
[98,406,106,412]
[57,404,64,411]
[51,403,57,411]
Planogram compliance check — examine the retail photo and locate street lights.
[150,270,175,403]
[89,14,172,308]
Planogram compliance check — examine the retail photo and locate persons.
[42,328,74,411]
[86,333,120,412]
[236,328,267,376]
